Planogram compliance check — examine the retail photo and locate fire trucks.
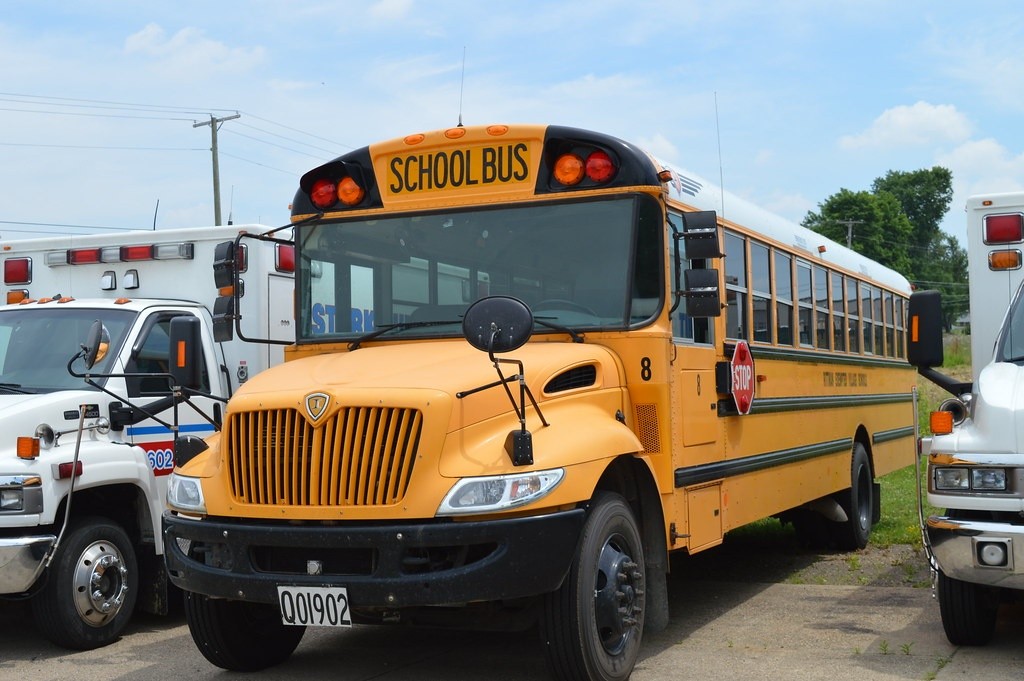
[915,190,1024,659]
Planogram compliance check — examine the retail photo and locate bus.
[159,119,925,672]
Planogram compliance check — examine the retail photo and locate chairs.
[411,304,468,322]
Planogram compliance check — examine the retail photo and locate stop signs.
[731,339,755,415]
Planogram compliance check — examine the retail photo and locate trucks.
[0,224,492,652]
